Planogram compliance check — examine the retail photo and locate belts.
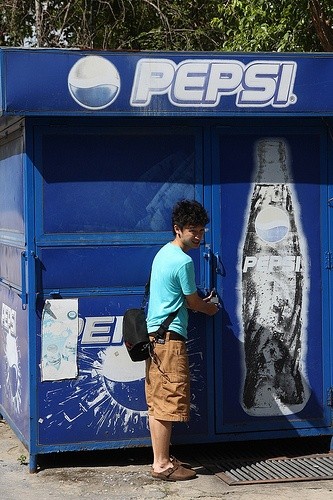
[149,331,184,340]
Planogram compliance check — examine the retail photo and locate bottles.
[241,140,306,416]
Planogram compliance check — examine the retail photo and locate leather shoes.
[171,458,191,468]
[151,465,197,480]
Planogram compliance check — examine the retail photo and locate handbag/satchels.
[121,308,152,363]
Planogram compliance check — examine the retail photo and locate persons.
[144,200,221,482]
